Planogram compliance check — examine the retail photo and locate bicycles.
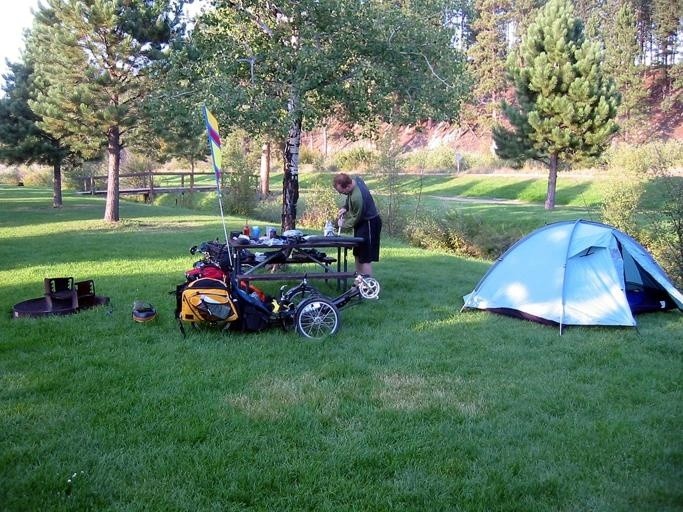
[278,273,380,339]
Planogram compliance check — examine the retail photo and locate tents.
[460,218,683,337]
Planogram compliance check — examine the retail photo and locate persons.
[332,173,381,279]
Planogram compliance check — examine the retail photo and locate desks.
[228,239,358,294]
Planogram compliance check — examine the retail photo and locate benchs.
[237,271,356,281]
[238,257,336,264]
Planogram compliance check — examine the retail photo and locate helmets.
[132,303,156,322]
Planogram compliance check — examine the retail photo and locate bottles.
[243,224,250,235]
[251,227,260,241]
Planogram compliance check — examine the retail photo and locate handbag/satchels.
[181,278,240,322]
